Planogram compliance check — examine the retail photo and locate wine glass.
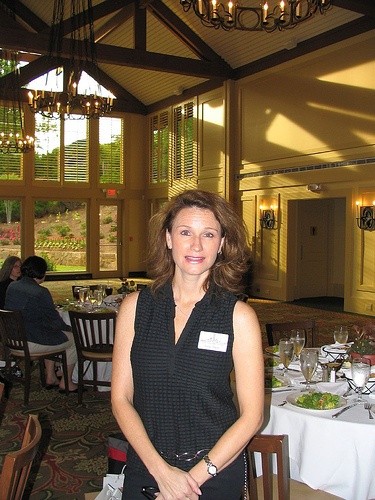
[73,284,115,312]
[280,325,349,391]
[352,357,372,406]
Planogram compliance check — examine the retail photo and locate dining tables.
[230,347,375,500]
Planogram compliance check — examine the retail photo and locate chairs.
[247,435,342,499]
[0,414,42,500]
[0,310,69,405]
[68,310,117,404]
[266,319,316,347]
[72,285,113,300]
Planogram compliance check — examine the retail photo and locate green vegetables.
[264,374,284,388]
[296,391,340,410]
[264,358,280,367]
[58,300,117,313]
[267,344,280,353]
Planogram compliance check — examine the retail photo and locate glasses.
[14,265,20,269]
[140,485,159,500]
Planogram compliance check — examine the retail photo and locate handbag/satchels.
[92,464,126,500]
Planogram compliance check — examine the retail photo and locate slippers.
[59,383,89,393]
[45,383,59,389]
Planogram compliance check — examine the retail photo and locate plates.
[265,376,291,393]
[287,390,346,410]
[264,345,281,356]
[263,358,279,372]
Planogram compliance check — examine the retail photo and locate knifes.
[333,403,358,418]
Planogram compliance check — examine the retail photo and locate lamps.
[260,205,275,229]
[0,50,34,154]
[28,0,112,120]
[180,0,333,33]
[356,201,375,231]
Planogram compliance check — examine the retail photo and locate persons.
[110,189,264,500]
[0,255,86,394]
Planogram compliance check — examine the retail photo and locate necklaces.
[175,304,190,318]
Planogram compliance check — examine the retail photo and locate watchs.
[203,455,217,477]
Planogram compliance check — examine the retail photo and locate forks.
[363,401,374,419]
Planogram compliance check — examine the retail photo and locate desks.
[55,307,119,391]
